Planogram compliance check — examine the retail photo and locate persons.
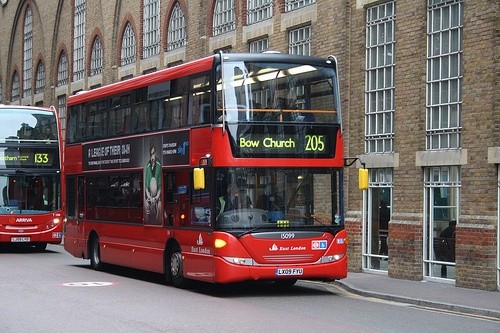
[224,182,281,211]
[142,139,163,226]
[378,198,390,261]
[247,96,295,133]
[38,127,55,139]
[440,218,456,262]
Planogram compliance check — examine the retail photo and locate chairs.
[433,237,454,277]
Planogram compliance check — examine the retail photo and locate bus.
[1,103,64,253]
[60,49,369,289]
